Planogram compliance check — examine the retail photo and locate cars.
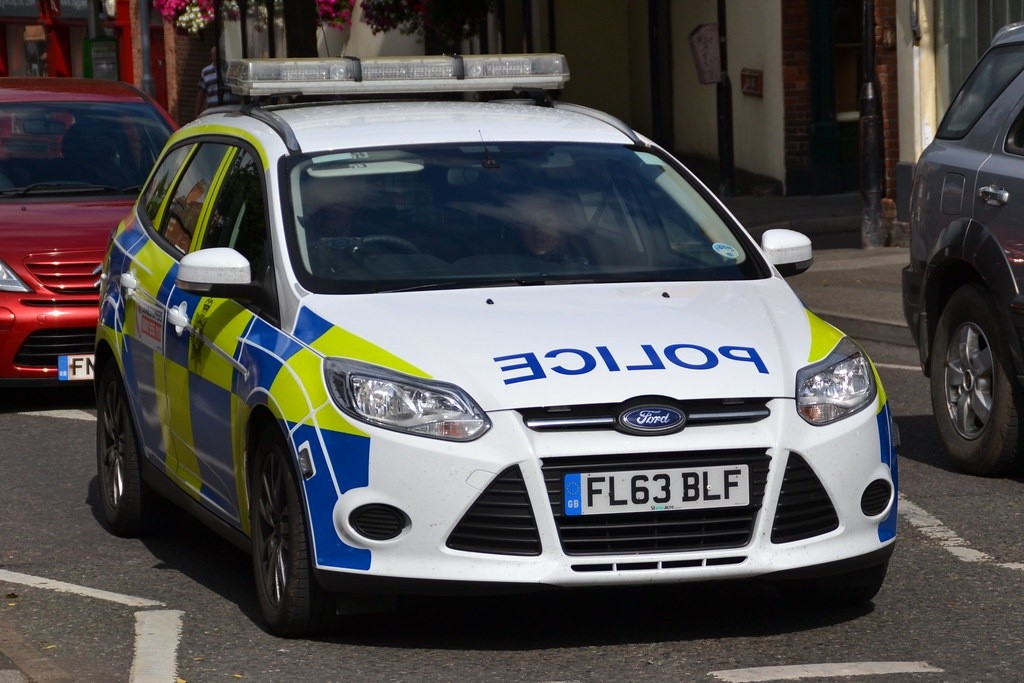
[94,54,899,638]
[0,77,180,385]
[43,318,89,331]
[900,20,1024,476]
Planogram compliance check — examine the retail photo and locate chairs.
[33,121,129,188]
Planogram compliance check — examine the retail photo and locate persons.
[507,191,585,269]
[194,46,230,118]
